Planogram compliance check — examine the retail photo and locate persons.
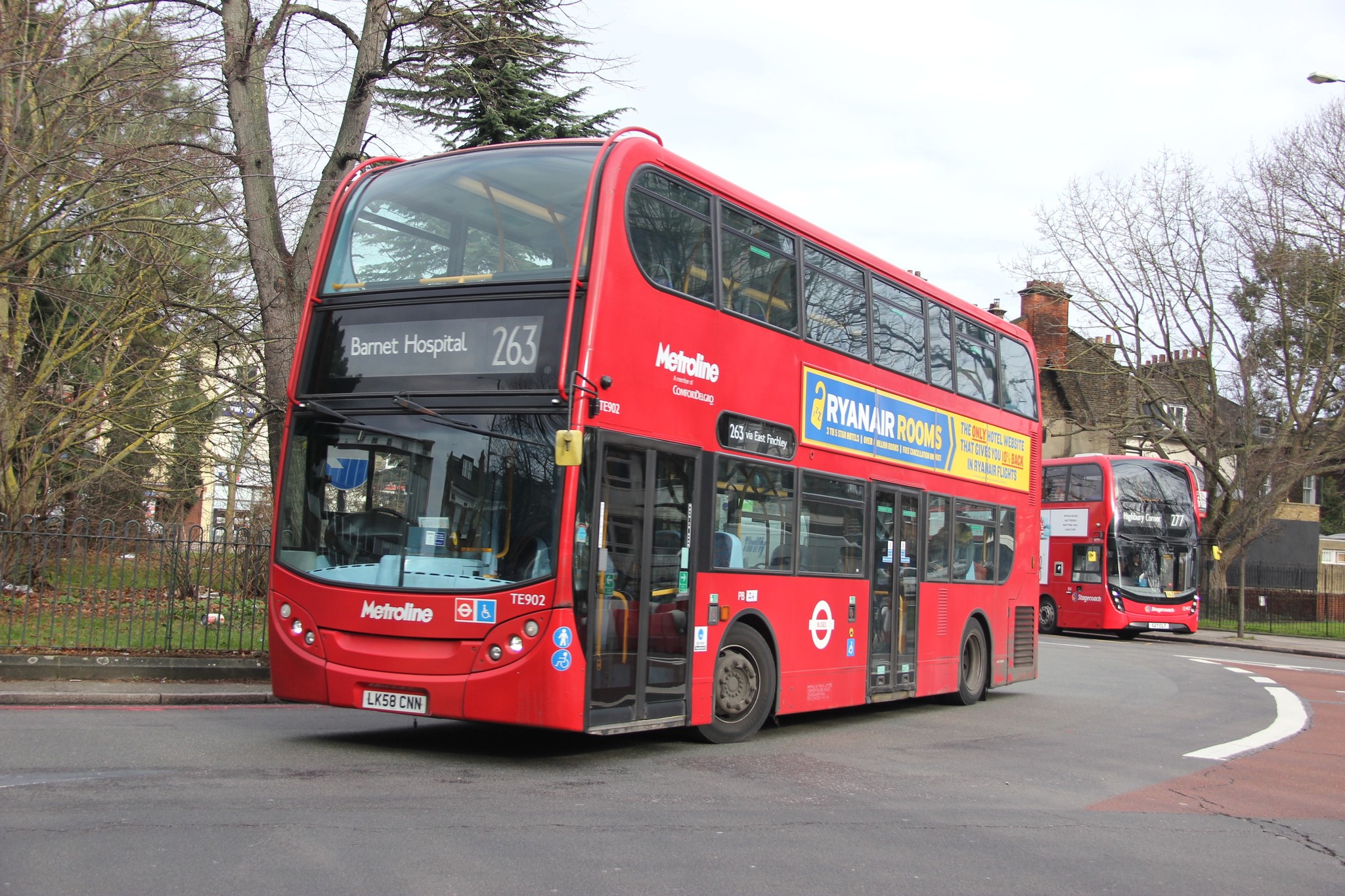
[928,510,975,579]
[1124,554,1143,578]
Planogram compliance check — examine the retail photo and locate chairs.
[715,531,829,574]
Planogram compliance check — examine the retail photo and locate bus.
[270,128,1040,736]
[882,454,1222,640]
